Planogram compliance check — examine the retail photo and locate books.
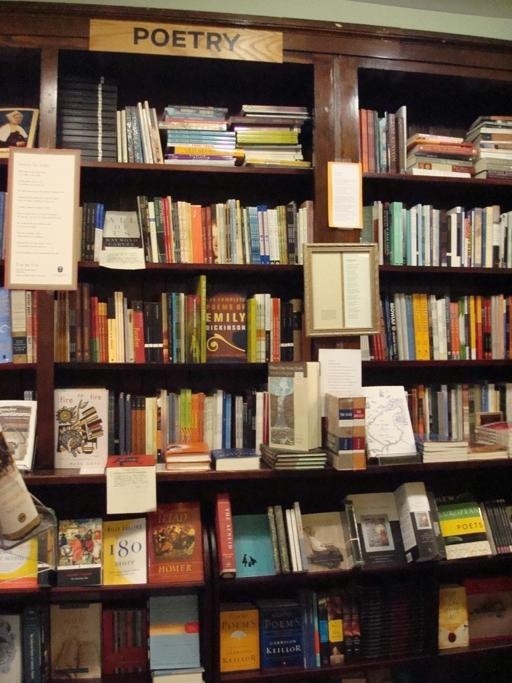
[0,68,512,682]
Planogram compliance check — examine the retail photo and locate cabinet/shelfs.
[0,1,512,683]
